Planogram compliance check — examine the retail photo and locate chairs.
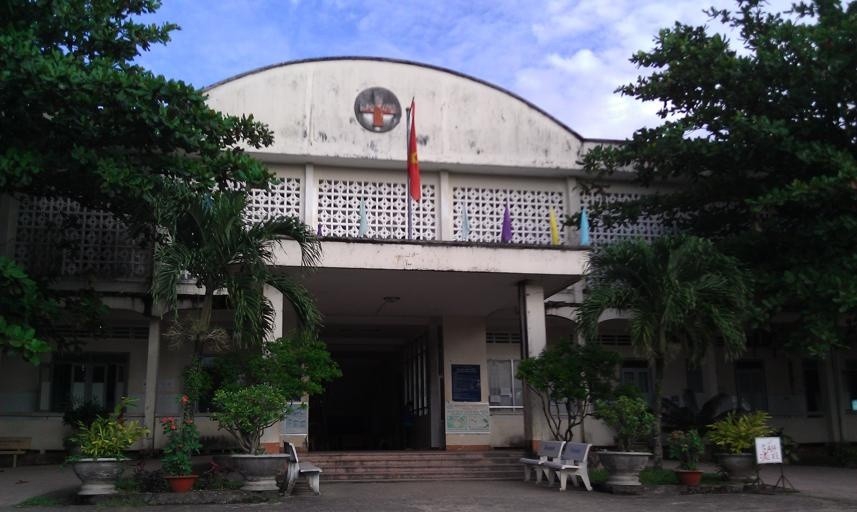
[280,441,321,496]
[519,440,593,490]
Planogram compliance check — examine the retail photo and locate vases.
[165,473,197,492]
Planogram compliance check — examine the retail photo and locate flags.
[580,205,593,248]
[548,204,560,246]
[461,198,472,240]
[359,190,370,239]
[407,98,424,203]
[499,200,514,244]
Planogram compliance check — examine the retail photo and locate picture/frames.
[282,402,308,434]
[444,362,489,434]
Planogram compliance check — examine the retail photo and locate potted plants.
[209,332,340,490]
[61,393,150,494]
[590,394,794,491]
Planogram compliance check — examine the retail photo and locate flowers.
[159,395,203,475]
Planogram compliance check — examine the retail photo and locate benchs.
[0,437,31,465]
